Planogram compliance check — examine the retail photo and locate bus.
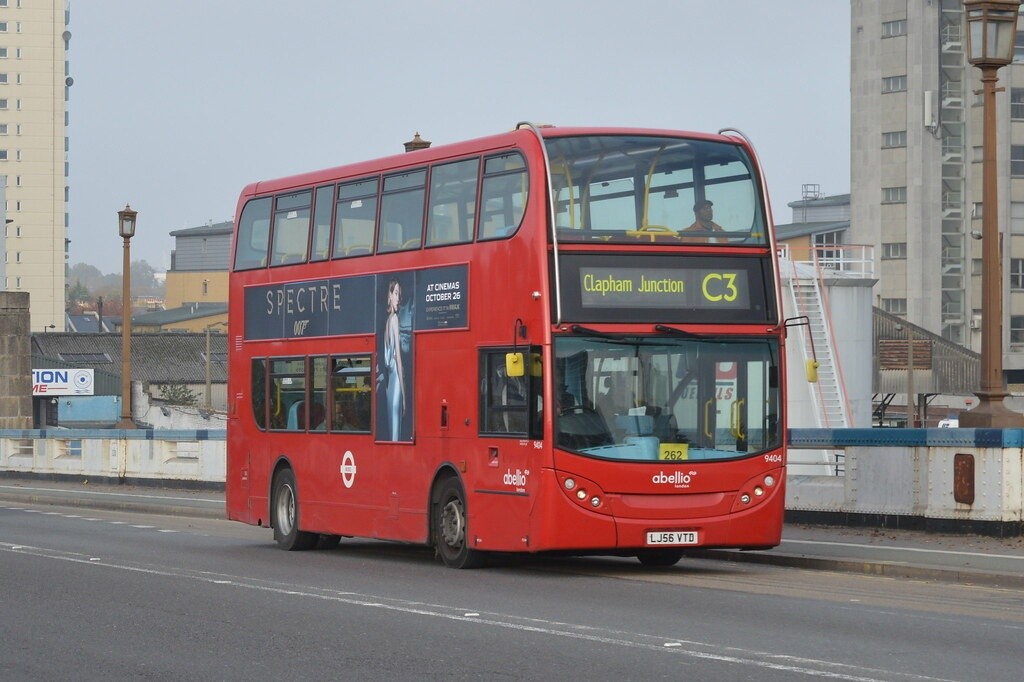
[223,122,820,571]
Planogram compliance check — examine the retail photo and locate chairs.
[287,400,325,429]
[653,415,690,443]
[259,224,675,272]
[558,405,615,451]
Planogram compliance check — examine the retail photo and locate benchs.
[277,390,337,416]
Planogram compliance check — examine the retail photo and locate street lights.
[958,1,1024,431]
[117,203,140,431]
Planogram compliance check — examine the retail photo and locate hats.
[693,199,713,212]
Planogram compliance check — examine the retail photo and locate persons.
[315,401,358,431]
[380,277,406,441]
[681,200,729,243]
